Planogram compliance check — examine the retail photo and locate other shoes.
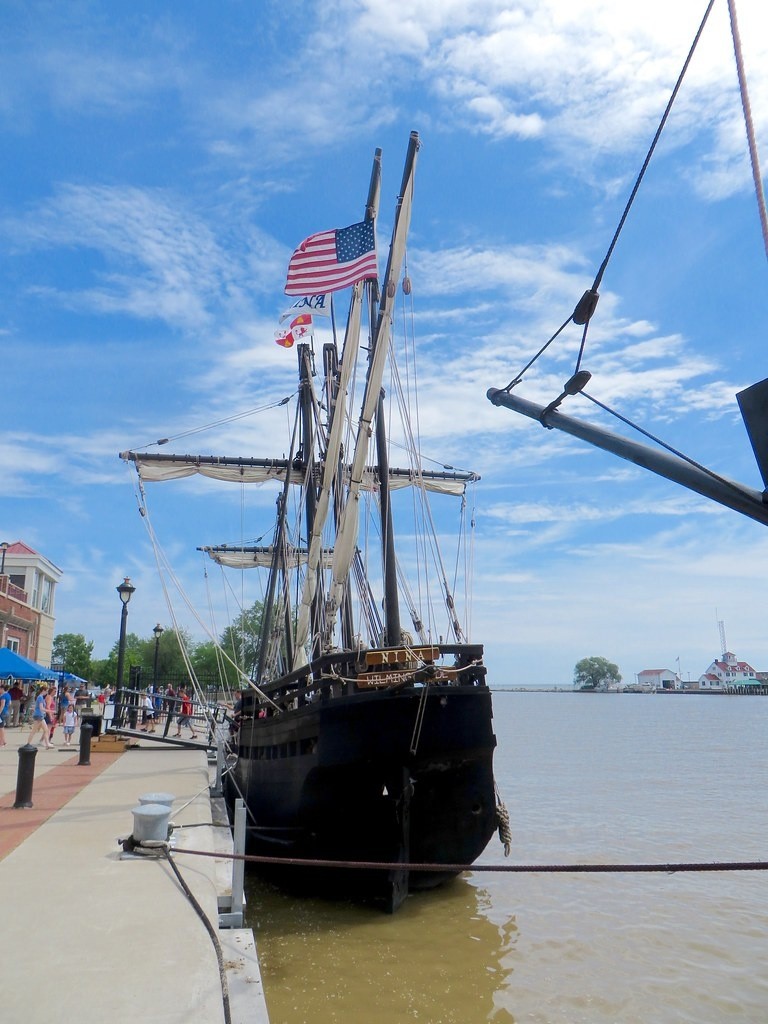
[148,730,155,733]
[190,735,198,739]
[172,734,181,737]
[141,728,147,732]
[64,741,71,746]
[47,745,54,749]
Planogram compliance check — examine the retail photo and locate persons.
[0,681,243,748]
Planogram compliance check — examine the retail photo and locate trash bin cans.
[81,714,103,736]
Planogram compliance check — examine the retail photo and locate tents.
[0,646,90,724]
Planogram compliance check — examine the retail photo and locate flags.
[273,314,312,348]
[279,292,331,325]
[283,218,377,299]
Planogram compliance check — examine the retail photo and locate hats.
[31,684,36,689]
[80,683,85,687]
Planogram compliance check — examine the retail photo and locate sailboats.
[120,131,496,914]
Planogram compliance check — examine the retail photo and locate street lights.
[152,624,163,694]
[111,576,137,728]
